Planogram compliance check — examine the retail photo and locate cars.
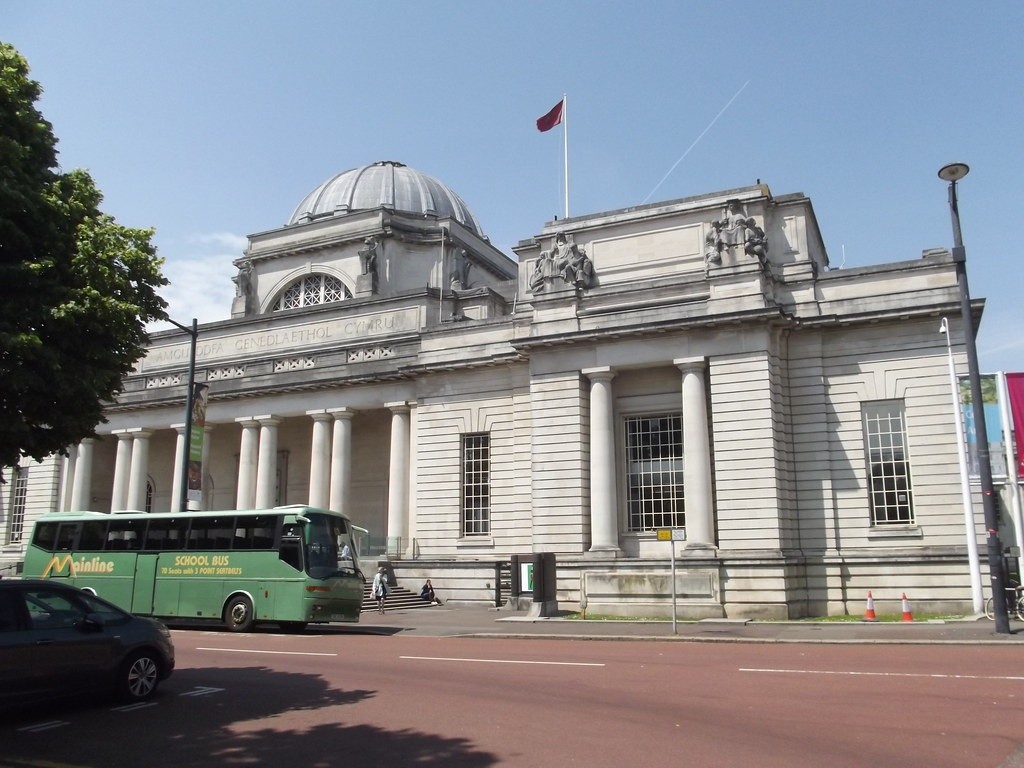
[0,576,178,704]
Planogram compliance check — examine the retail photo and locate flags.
[536,99,564,132]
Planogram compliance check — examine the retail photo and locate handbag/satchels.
[370,592,376,600]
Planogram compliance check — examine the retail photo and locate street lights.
[934,160,1012,634]
[156,308,200,510]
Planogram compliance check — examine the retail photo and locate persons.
[701,200,770,261]
[362,236,379,276]
[235,263,254,297]
[340,542,351,560]
[529,232,593,290]
[421,579,443,606]
[372,567,393,615]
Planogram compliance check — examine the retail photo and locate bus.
[18,504,370,633]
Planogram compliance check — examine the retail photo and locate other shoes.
[431,602,438,605]
[379,610,384,615]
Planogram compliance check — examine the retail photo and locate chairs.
[99,536,274,550]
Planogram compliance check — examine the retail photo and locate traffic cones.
[900,592,914,621]
[861,589,879,621]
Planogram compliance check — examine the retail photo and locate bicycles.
[984,585,1024,622]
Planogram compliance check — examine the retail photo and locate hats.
[377,567,387,572]
